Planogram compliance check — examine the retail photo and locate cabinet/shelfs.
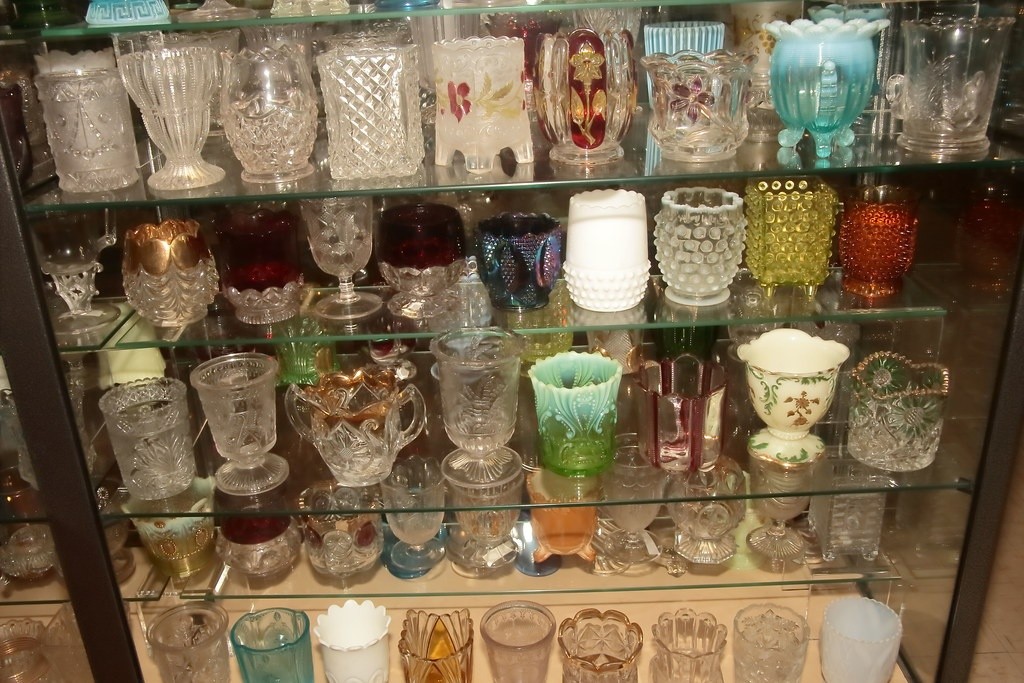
[0,0,1024,683]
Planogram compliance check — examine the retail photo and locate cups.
[528,353,622,478]
[1,424,55,576]
[808,452,886,561]
[99,377,196,500]
[432,36,534,174]
[563,189,651,312]
[147,596,903,683]
[848,351,950,472]
[640,50,748,162]
[533,28,637,165]
[474,212,562,312]
[113,478,385,579]
[634,354,727,473]
[762,18,890,158]
[123,208,301,327]
[284,366,426,486]
[316,45,425,179]
[653,187,747,306]
[897,17,1016,154]
[34,48,140,193]
[220,45,318,183]
[745,177,919,302]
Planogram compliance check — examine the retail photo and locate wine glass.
[449,472,524,569]
[23,208,120,334]
[429,326,528,489]
[190,353,289,496]
[117,47,225,190]
[747,457,810,558]
[665,459,746,563]
[298,195,383,320]
[737,329,850,468]
[603,446,664,564]
[377,204,466,319]
[381,454,446,569]
